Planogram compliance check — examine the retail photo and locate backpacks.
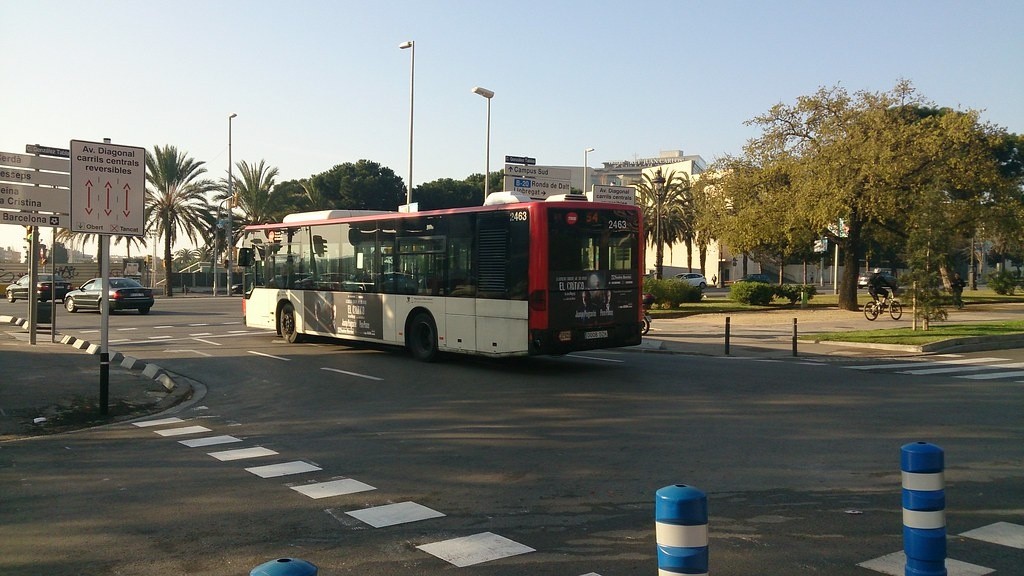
[868,276,879,296]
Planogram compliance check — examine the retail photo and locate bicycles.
[863,289,903,321]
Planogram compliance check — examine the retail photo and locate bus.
[237,194,644,363]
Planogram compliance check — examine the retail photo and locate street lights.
[583,147,595,197]
[474,88,495,200]
[228,113,237,296]
[398,41,415,203]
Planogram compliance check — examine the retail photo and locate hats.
[874,267,881,274]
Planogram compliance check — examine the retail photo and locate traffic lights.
[234,192,242,208]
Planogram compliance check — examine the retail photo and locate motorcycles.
[642,294,653,335]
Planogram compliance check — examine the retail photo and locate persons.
[951,272,966,310]
[711,274,717,285]
[810,272,814,283]
[867,268,894,305]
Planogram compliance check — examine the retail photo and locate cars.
[857,271,898,289]
[232,283,244,294]
[6,273,73,305]
[266,273,419,293]
[672,273,707,289]
[63,276,155,315]
